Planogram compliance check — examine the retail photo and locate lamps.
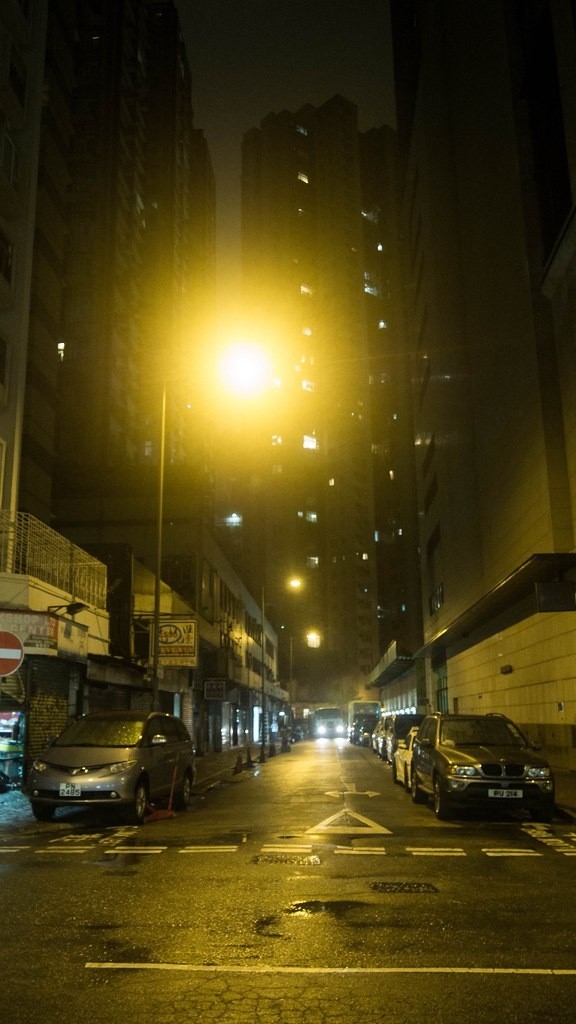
[47,602,88,616]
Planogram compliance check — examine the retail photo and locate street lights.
[289,633,319,735]
[152,357,275,709]
[261,579,301,764]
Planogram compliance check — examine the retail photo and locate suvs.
[410,711,556,820]
[372,714,426,766]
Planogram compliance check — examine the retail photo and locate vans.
[27,710,194,822]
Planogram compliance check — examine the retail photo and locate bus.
[314,706,343,738]
[346,700,380,737]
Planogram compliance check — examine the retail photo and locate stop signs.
[0,631,25,677]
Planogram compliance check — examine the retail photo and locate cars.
[394,726,419,792]
[351,721,376,747]
